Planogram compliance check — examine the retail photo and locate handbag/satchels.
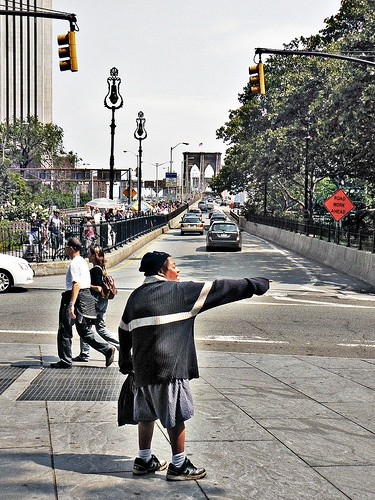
[117,371,139,427]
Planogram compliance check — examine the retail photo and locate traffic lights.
[249,62,266,95]
[57,31,79,72]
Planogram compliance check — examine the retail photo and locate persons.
[69,247,126,363]
[48,237,118,370]
[9,193,184,265]
[119,250,274,480]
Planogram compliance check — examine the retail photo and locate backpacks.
[94,264,118,301]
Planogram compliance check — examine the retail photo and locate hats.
[139,251,169,277]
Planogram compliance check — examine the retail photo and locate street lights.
[169,142,190,194]
[156,160,174,197]
[2,123,30,161]
[134,110,147,214]
[123,150,139,200]
[103,66,123,213]
[180,157,195,203]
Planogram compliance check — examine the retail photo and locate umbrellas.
[85,198,120,217]
[129,199,153,214]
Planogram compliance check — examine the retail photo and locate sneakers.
[132,453,168,475]
[166,456,207,481]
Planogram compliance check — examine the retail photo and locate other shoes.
[50,363,72,369]
[105,346,116,367]
[71,356,89,362]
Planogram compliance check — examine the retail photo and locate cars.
[0,253,36,294]
[179,193,246,236]
[204,220,246,251]
